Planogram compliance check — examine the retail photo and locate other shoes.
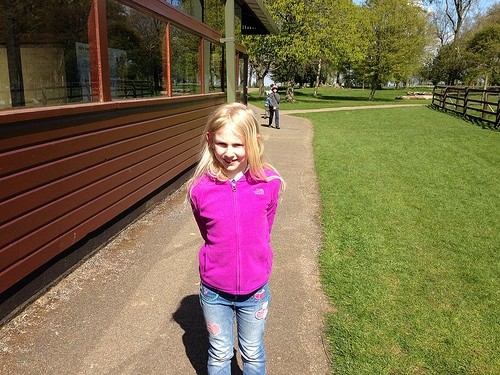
[269,125,280,129]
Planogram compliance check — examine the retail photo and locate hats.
[272,86,278,89]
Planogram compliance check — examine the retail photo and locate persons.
[182,103,288,375]
[264,84,276,119]
[267,85,281,129]
